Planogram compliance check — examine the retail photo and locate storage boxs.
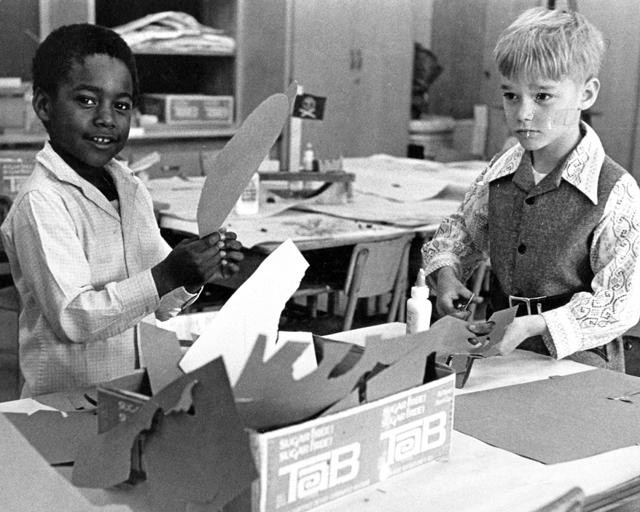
[138,93,233,124]
[95,335,457,512]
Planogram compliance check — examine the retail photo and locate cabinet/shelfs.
[0,0,243,145]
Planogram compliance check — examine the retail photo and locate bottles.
[405,269,433,335]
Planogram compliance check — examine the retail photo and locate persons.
[419,6,639,373]
[1,22,243,399]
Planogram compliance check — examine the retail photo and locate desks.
[146,177,439,326]
[0,321,640,512]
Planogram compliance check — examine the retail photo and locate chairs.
[281,229,418,338]
